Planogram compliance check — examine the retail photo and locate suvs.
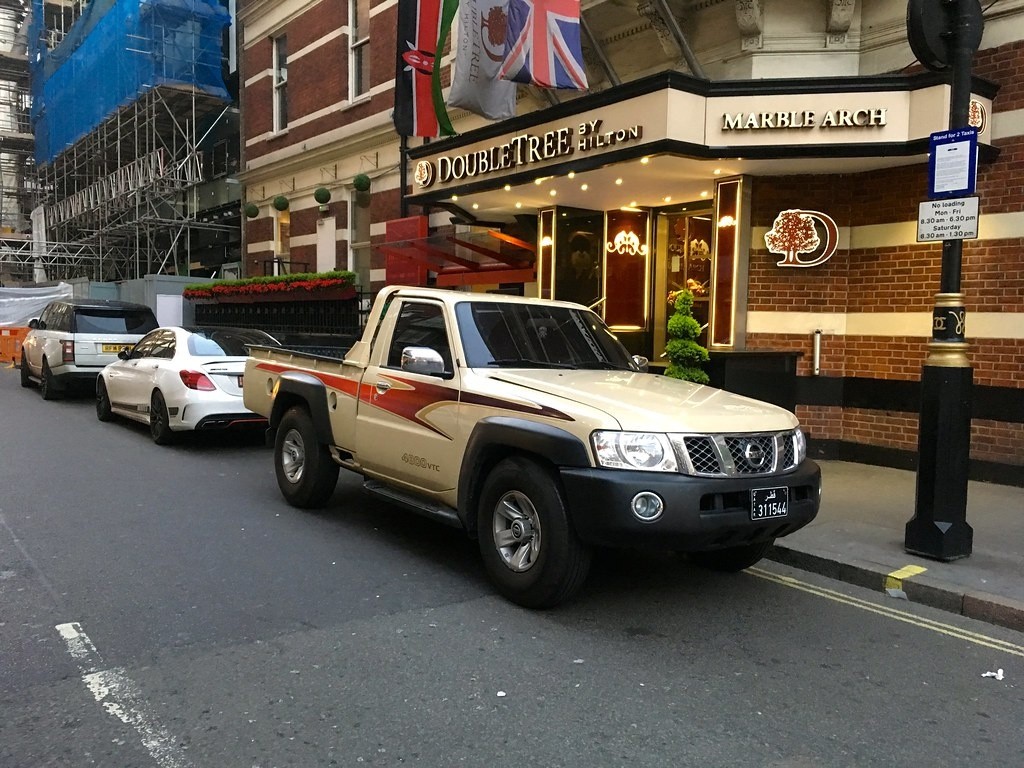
[19,297,160,400]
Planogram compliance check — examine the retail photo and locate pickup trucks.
[242,286,822,610]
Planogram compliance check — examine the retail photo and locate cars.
[94,325,282,445]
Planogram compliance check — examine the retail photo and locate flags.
[395,1,589,139]
